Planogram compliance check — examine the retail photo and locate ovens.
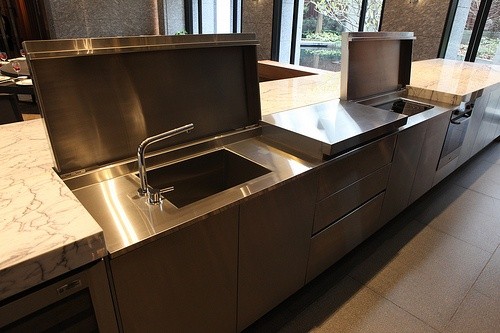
[437,103,475,169]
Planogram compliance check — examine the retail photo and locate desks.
[0,55,38,114]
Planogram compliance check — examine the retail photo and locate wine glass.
[0,52,7,62]
[12,61,21,78]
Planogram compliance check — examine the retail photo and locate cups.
[20,49,26,57]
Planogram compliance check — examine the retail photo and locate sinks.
[373,98,434,118]
[134,148,271,209]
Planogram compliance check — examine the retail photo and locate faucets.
[137,123,195,192]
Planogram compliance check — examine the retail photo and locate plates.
[16,78,33,85]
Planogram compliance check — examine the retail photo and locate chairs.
[0,93,24,125]
